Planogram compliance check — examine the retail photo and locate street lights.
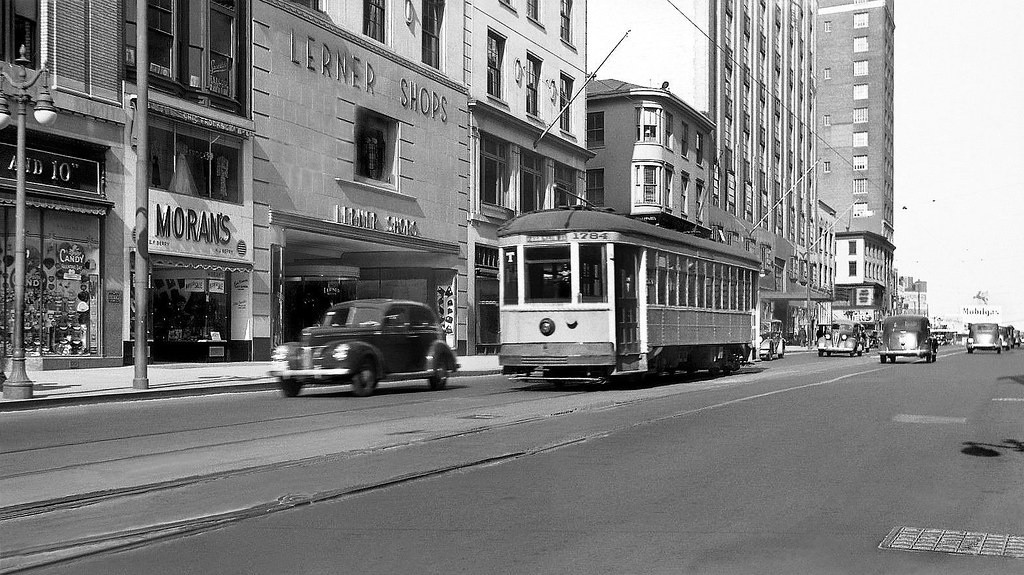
[0,44,59,400]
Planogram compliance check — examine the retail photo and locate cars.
[998,325,1024,351]
[267,296,462,399]
[965,322,1003,354]
[876,315,939,363]
[815,319,867,357]
[759,318,788,361]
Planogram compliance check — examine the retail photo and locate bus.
[492,148,765,389]
[831,281,887,348]
